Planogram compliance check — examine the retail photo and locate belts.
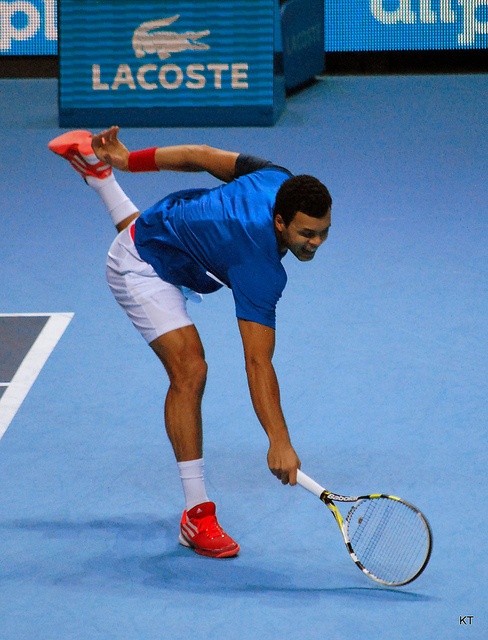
[130,221,136,245]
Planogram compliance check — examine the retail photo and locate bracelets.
[126,147,160,173]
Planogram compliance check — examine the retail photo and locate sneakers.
[48,129,112,185]
[178,501,240,558]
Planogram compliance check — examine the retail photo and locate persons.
[47,126,331,557]
